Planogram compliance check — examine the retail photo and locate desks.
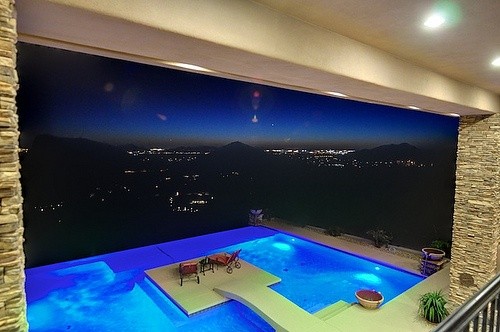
[198,258,217,275]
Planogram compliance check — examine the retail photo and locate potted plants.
[328,224,346,237]
[417,289,450,324]
[433,240,449,250]
[366,228,394,248]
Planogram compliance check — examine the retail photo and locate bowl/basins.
[355,289,384,310]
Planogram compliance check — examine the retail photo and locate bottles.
[205,254,208,263]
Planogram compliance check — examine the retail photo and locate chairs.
[207,248,243,274]
[179,262,199,286]
